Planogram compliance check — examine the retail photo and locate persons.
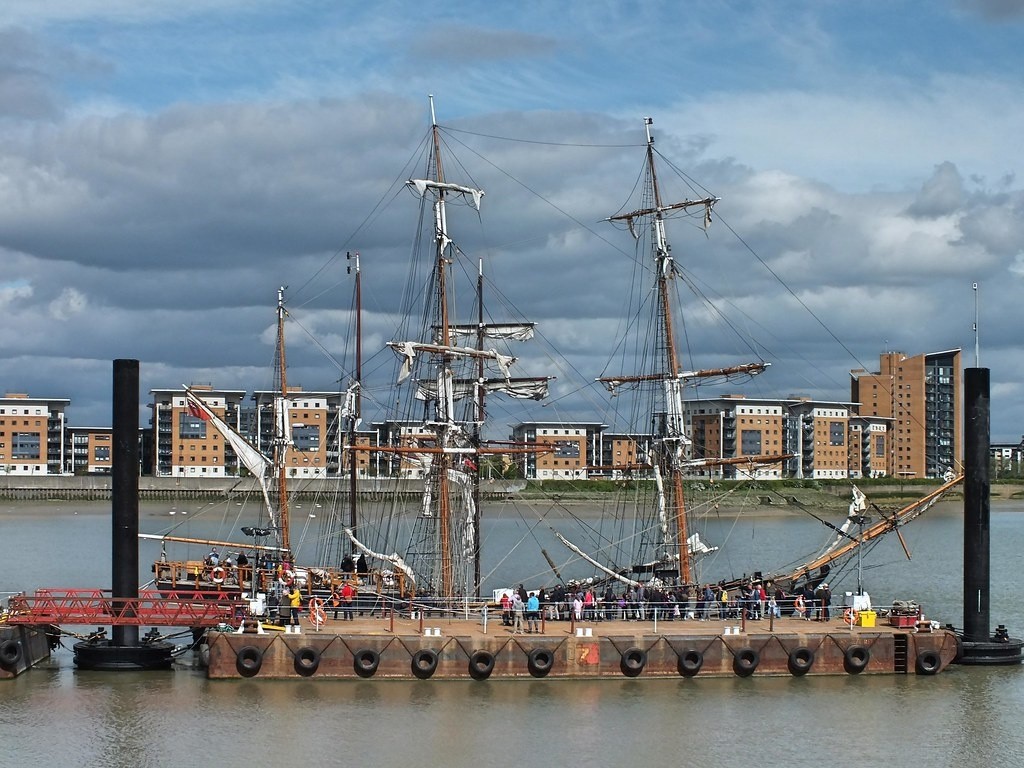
[203,547,371,628]
[512,595,526,635]
[526,592,539,633]
[499,580,832,626]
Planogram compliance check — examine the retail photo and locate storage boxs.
[857,611,877,627]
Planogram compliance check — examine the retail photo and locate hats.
[817,584,824,589]
[334,587,338,590]
[513,589,519,593]
[668,591,674,595]
[822,583,828,587]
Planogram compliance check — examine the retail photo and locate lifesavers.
[844,608,859,625]
[308,596,328,625]
[843,645,870,669]
[0,639,24,666]
[916,649,942,675]
[413,647,438,673]
[354,646,381,671]
[734,646,762,671]
[620,647,647,671]
[469,649,495,673]
[529,646,554,673]
[295,644,321,668]
[209,566,226,584]
[679,648,704,671]
[277,569,295,586]
[787,645,815,670]
[794,598,806,612]
[236,645,263,671]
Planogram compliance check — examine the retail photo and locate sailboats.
[135,90,967,621]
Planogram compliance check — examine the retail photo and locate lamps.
[241,527,286,537]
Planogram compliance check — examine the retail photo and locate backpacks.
[610,592,616,600]
[708,591,714,601]
[720,589,728,602]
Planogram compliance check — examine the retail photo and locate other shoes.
[526,631,532,634]
[699,619,705,622]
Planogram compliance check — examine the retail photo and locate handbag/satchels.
[345,596,353,603]
[643,589,650,600]
[298,605,303,612]
